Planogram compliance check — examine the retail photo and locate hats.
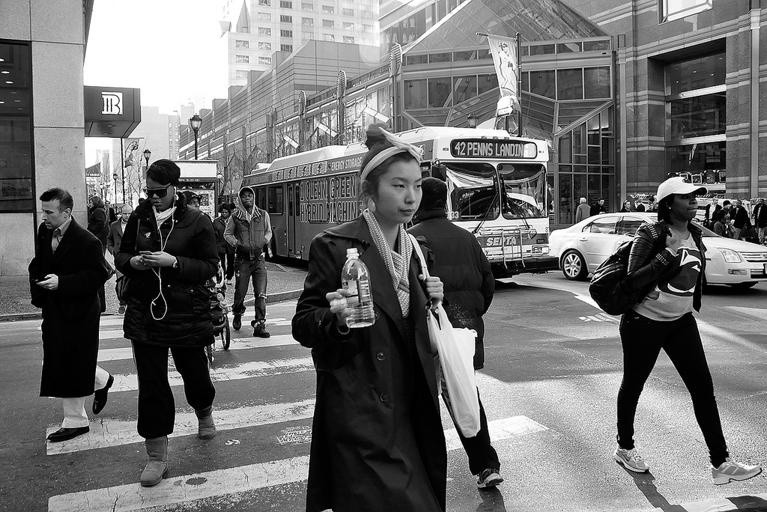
[420,177,448,209]
[657,177,708,204]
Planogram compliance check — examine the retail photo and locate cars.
[546,210,767,290]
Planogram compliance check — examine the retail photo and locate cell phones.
[139,251,152,254]
[34,278,49,282]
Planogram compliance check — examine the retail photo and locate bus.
[238,124,555,287]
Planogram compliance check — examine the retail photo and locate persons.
[620,195,658,212]
[29,189,113,443]
[114,159,219,486]
[590,198,606,216]
[185,186,239,286]
[405,178,504,490]
[612,176,762,485]
[705,196,767,244]
[88,196,135,315]
[223,186,273,338]
[291,121,447,512]
[575,196,591,223]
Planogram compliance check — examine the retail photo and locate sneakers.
[614,447,649,473]
[233,313,242,330]
[710,457,762,485]
[254,324,270,338]
[477,469,504,488]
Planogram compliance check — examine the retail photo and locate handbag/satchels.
[590,241,657,315]
[115,269,135,304]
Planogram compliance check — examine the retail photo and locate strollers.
[201,263,233,353]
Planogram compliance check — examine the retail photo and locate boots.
[140,435,168,486]
[196,405,216,439]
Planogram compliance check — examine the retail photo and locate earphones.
[174,186,176,193]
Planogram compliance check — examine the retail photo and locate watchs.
[172,257,180,271]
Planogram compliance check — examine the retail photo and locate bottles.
[339,247,375,329]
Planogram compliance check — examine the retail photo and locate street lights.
[98,183,104,199]
[112,172,120,204]
[188,112,203,159]
[141,149,151,168]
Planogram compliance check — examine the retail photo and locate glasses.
[144,183,170,198]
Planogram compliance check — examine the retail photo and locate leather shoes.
[48,427,89,442]
[93,373,114,413]
